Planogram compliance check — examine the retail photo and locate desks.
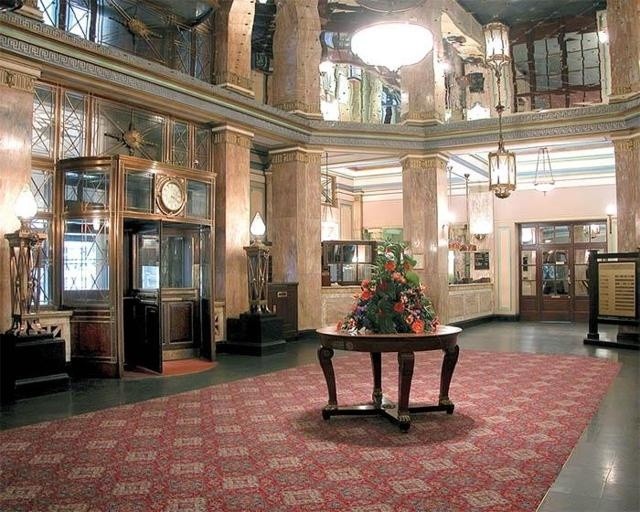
[316,324,462,436]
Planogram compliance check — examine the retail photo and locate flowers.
[335,238,443,336]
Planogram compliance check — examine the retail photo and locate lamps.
[12,182,41,233]
[482,15,512,108]
[532,146,554,193]
[447,166,461,250]
[249,210,267,245]
[605,202,618,234]
[488,108,516,202]
[471,207,492,241]
[321,153,342,243]
[347,17,435,75]
[461,173,477,252]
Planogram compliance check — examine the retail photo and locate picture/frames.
[411,253,427,271]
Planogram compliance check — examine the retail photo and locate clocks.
[154,175,187,217]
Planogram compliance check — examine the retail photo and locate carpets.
[122,359,219,381]
[0,347,624,512]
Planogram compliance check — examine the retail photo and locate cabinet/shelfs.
[268,281,301,337]
[321,239,377,286]
